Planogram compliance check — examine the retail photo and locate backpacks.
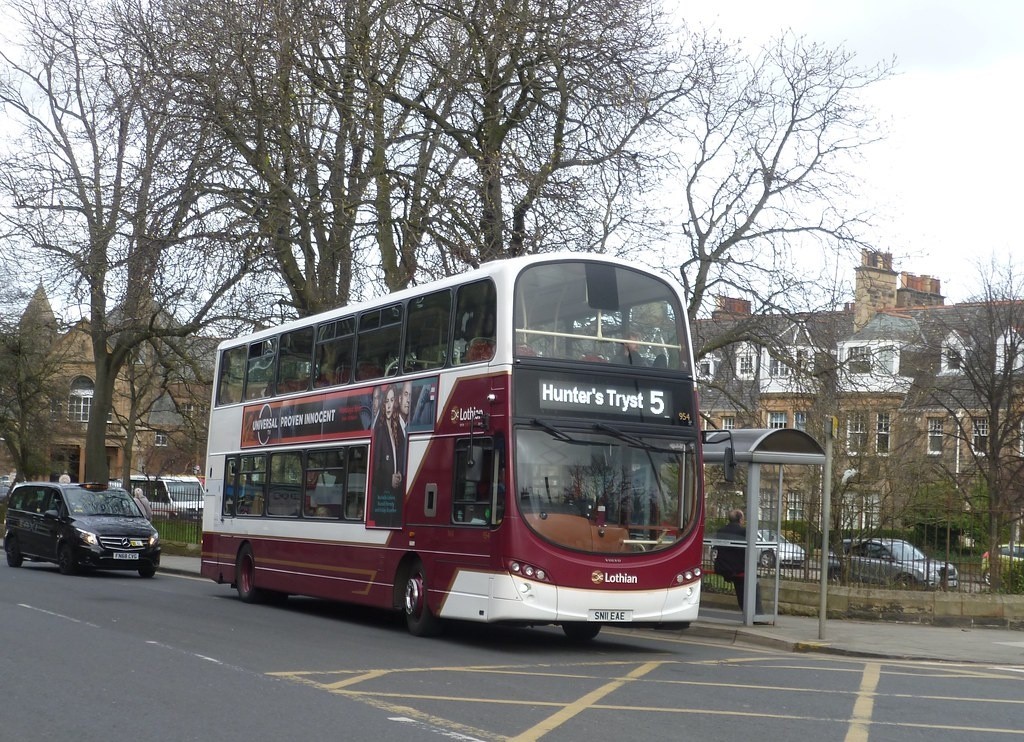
[714,552,737,582]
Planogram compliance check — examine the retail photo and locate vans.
[2,481,161,578]
[129,476,207,520]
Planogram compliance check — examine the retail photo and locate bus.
[202,253,738,644]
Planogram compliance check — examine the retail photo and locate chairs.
[277,336,611,396]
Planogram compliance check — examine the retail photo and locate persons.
[133,488,153,520]
[318,364,336,388]
[367,385,383,433]
[714,508,778,626]
[263,380,274,397]
[314,506,333,517]
[618,334,648,367]
[395,380,413,459]
[250,491,265,515]
[355,505,365,520]
[495,464,507,497]
[370,383,407,531]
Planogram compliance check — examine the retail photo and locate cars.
[711,528,806,569]
[825,537,961,593]
[0,473,28,499]
[979,544,1024,587]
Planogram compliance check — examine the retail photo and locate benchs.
[698,569,758,623]
